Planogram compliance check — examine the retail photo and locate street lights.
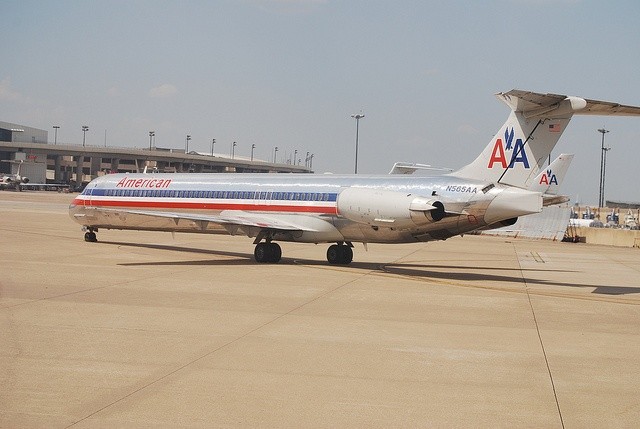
[597,128,609,207]
[307,151,309,167]
[82,126,88,146]
[602,147,611,207]
[274,146,278,163]
[212,139,215,156]
[251,144,255,161]
[351,113,365,174]
[233,141,236,158]
[294,149,298,165]
[69,88,640,263]
[311,153,314,167]
[149,131,155,151]
[187,135,191,154]
[53,126,60,144]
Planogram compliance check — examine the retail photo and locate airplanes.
[0,159,29,191]
